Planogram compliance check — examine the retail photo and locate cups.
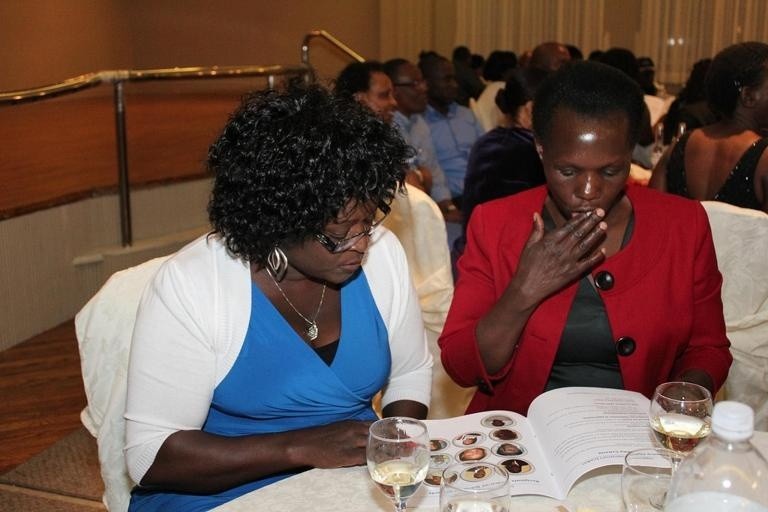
[620,445,689,511]
[437,459,512,512]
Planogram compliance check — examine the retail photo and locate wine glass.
[649,380,714,507]
[364,416,431,512]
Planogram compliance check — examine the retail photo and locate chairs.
[378,180,475,419]
[74,253,180,512]
[694,201,768,432]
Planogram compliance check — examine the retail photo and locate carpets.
[0,426,104,512]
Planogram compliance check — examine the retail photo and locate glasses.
[305,198,392,255]
[393,77,427,87]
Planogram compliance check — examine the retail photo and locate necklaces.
[265,267,327,341]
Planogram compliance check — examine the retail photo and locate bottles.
[675,122,687,143]
[662,399,768,512]
[650,121,664,172]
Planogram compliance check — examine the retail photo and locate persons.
[337,41,584,278]
[125,87,433,511]
[590,41,768,216]
[425,416,528,485]
[438,63,733,417]
